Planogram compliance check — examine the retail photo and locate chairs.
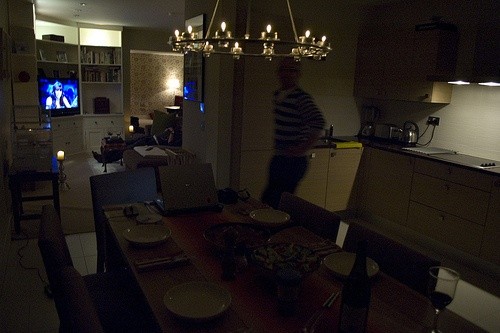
[34,163,440,333]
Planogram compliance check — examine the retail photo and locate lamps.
[57,150,65,160]
[167,0,333,64]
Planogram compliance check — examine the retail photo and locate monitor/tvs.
[39,77,80,117]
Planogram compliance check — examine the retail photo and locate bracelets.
[171,130,174,133]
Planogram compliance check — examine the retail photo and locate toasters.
[375,124,398,138]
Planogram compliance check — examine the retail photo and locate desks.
[9,159,61,236]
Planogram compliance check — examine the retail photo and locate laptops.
[153,163,218,217]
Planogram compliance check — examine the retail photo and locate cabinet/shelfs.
[0,1,125,166]
[295,147,500,282]
[352,20,457,105]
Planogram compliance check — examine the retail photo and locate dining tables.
[99,195,490,333]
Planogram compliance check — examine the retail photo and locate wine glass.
[421,266,460,333]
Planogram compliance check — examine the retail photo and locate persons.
[263,57,326,208]
[92,111,183,163]
[46,81,71,110]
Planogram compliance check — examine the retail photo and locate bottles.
[336,255,372,333]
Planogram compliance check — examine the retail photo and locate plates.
[122,223,172,246]
[136,212,165,225]
[324,254,378,276]
[202,222,272,251]
[251,241,321,276]
[162,281,233,319]
[249,209,290,226]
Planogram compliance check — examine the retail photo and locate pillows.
[151,111,178,137]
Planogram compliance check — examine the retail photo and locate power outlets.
[428,117,440,125]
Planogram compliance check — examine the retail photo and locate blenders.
[360,104,380,136]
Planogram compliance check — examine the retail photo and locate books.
[80,46,121,64]
[81,66,121,82]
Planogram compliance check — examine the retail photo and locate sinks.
[326,138,362,148]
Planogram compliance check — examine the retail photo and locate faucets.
[323,123,334,137]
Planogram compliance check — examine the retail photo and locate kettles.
[397,121,419,145]
